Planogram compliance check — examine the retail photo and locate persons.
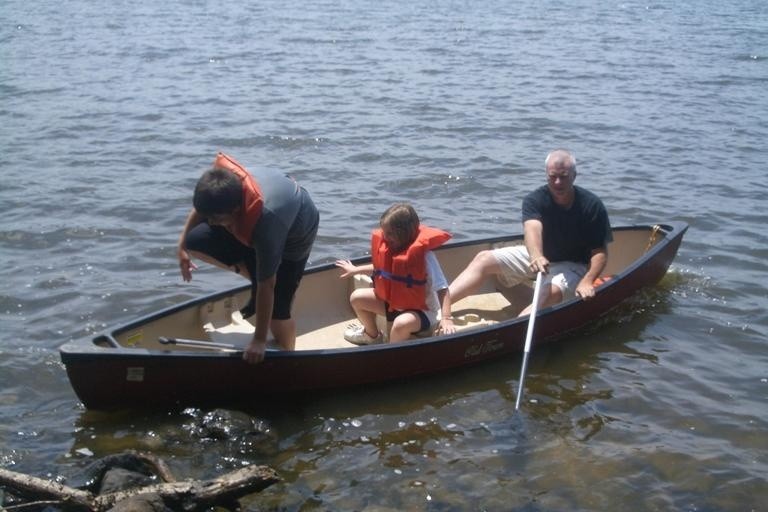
[447,148,615,319]
[335,202,455,348]
[176,150,319,367]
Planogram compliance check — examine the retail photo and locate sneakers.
[344,328,384,345]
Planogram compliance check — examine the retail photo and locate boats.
[56,219,690,407]
